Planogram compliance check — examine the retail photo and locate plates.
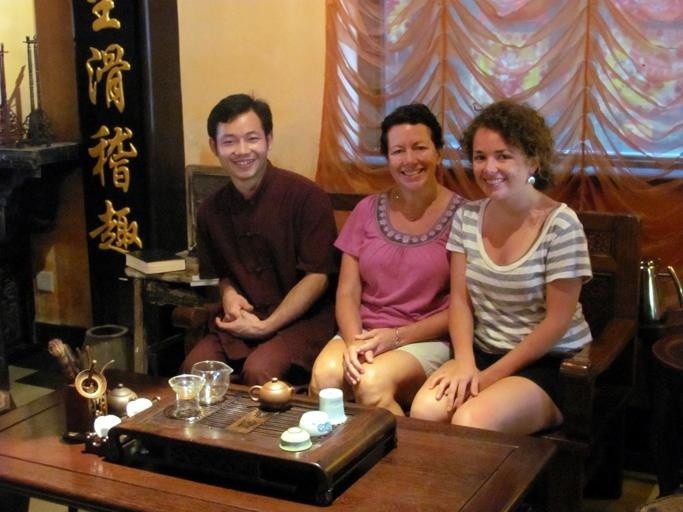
[279,425,313,453]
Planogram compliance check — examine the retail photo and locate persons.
[306,101,470,417]
[173,90,340,387]
[410,98,593,437]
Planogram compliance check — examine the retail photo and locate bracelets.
[392,328,400,347]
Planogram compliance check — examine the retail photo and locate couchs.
[171,192,641,501]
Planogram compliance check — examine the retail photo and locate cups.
[189,360,235,405]
[299,409,335,438]
[318,387,348,424]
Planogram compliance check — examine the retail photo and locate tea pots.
[248,376,297,411]
[105,383,139,418]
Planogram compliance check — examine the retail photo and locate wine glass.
[168,372,206,420]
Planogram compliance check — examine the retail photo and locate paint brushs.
[48,339,117,387]
